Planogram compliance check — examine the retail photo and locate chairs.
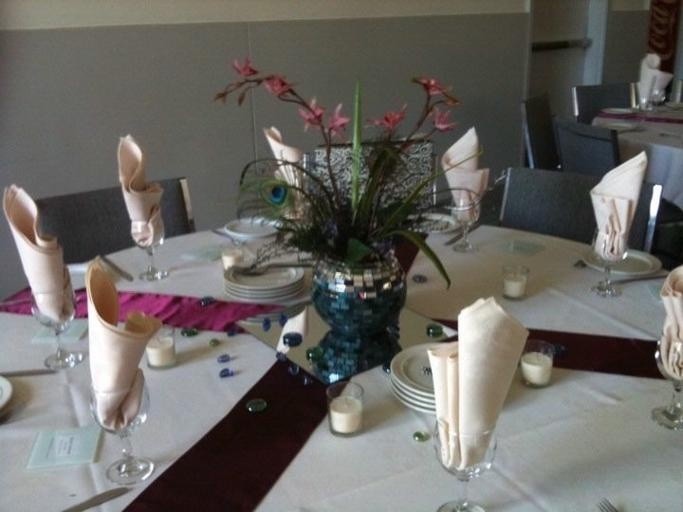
[31,171,198,265]
[497,80,681,277]
[306,136,439,218]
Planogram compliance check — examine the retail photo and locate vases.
[302,244,410,379]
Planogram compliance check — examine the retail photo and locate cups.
[219,247,240,269]
[144,325,177,370]
[502,263,529,301]
[518,339,554,388]
[326,381,366,436]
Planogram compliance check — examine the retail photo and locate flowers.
[209,51,489,294]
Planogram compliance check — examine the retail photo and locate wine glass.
[590,228,628,299]
[637,88,666,129]
[28,284,83,369]
[449,197,481,253]
[651,330,682,431]
[433,421,499,512]
[131,220,167,279]
[88,370,154,484]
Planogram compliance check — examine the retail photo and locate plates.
[601,123,631,133]
[225,260,306,323]
[581,247,662,277]
[224,218,277,240]
[388,342,440,414]
[409,213,460,235]
[0,374,13,413]
[65,262,89,290]
[601,107,631,114]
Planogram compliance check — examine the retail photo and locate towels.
[438,115,493,224]
[256,120,317,227]
[112,130,170,247]
[2,183,76,321]
[82,250,168,433]
[653,261,682,383]
[635,50,674,107]
[423,291,530,480]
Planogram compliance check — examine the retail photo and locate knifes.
[62,485,130,512]
[97,252,134,284]
[610,274,667,287]
[446,221,482,247]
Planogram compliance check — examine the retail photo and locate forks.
[597,498,617,510]
